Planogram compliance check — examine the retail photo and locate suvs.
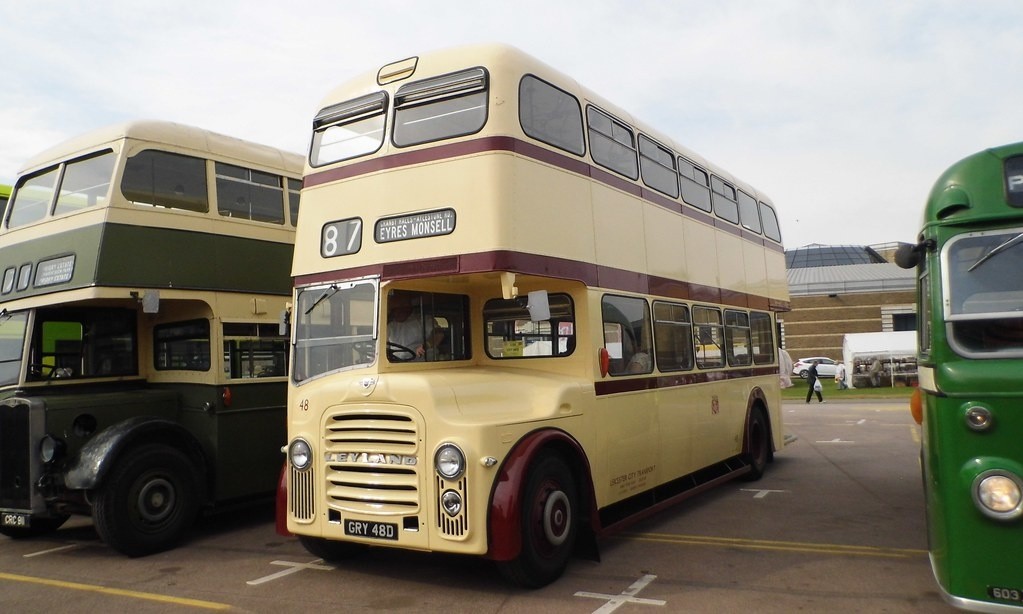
[793,357,845,379]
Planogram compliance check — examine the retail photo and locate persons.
[387,292,445,361]
[805,361,826,404]
[622,329,649,372]
[835,360,847,389]
[870,358,881,387]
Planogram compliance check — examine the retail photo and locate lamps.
[829,294,837,297]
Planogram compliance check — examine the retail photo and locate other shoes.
[819,401,826,404]
[807,401,810,404]
[872,385,880,388]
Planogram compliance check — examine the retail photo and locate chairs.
[218,206,235,217]
[92,332,122,375]
[664,354,761,370]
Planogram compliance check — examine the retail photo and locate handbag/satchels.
[814,377,822,391]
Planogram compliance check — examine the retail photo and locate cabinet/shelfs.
[854,358,918,389]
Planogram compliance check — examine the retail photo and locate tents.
[842,330,917,389]
[523,340,567,355]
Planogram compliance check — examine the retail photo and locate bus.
[278,40,790,585]
[0,184,102,379]
[0,119,379,556]
[896,141,1023,614]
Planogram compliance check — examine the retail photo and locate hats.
[388,296,409,311]
